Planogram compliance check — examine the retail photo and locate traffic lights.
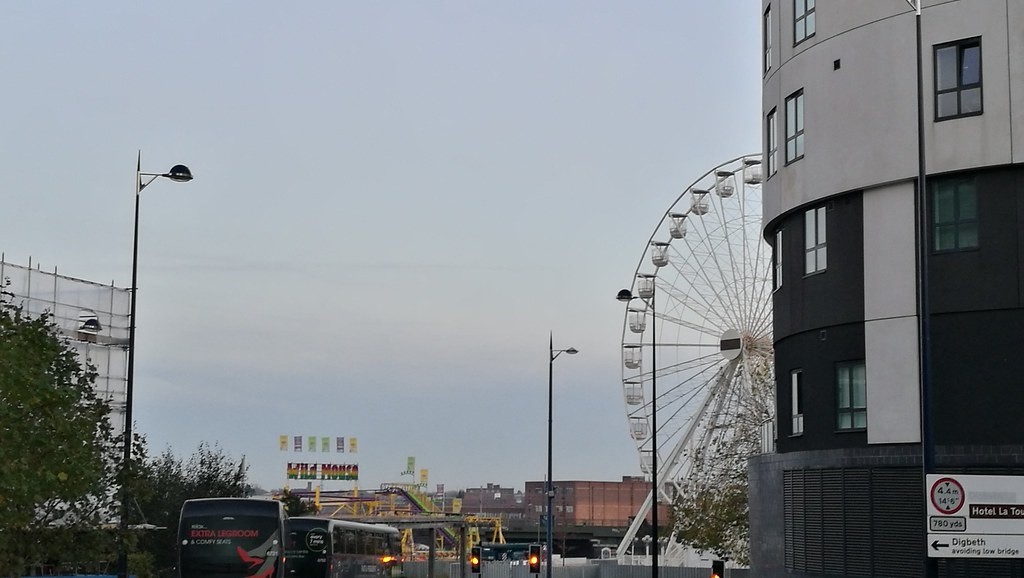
[528,544,541,574]
[471,547,481,574]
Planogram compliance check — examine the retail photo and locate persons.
[502,548,513,563]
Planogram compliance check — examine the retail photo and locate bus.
[174,497,406,578]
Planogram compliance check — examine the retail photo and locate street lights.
[617,280,657,578]
[546,330,579,578]
[115,149,193,578]
